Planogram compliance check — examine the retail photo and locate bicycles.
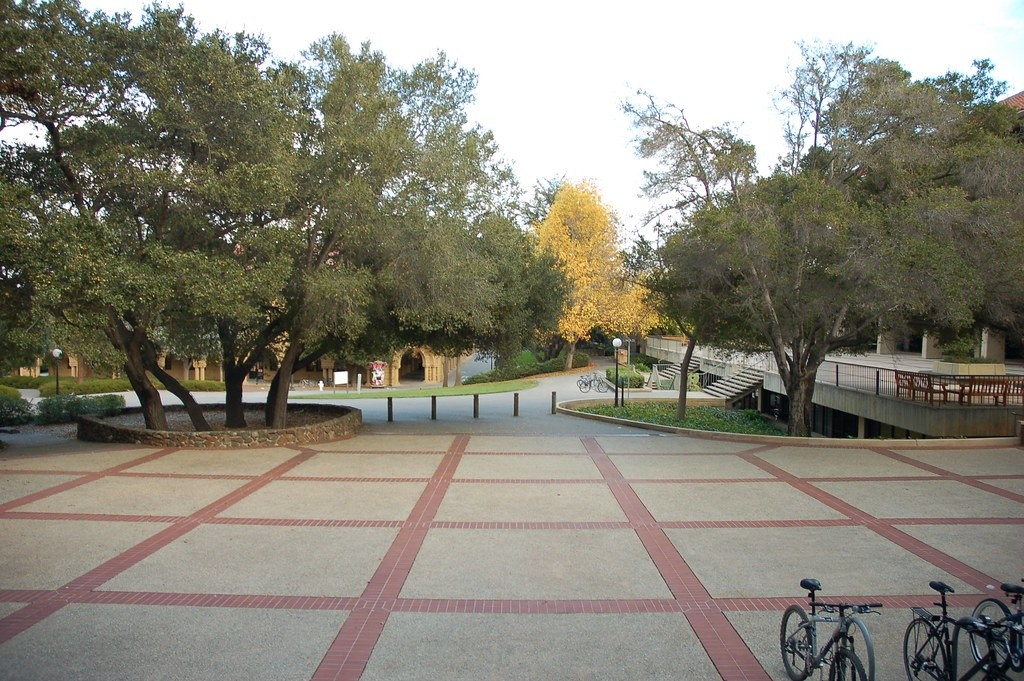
[968,577,1024,674]
[300,376,334,386]
[577,371,609,393]
[902,578,1013,681]
[779,578,883,681]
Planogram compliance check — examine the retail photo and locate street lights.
[53,349,63,396]
[612,338,622,408]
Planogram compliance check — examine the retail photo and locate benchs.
[894,369,1024,407]
